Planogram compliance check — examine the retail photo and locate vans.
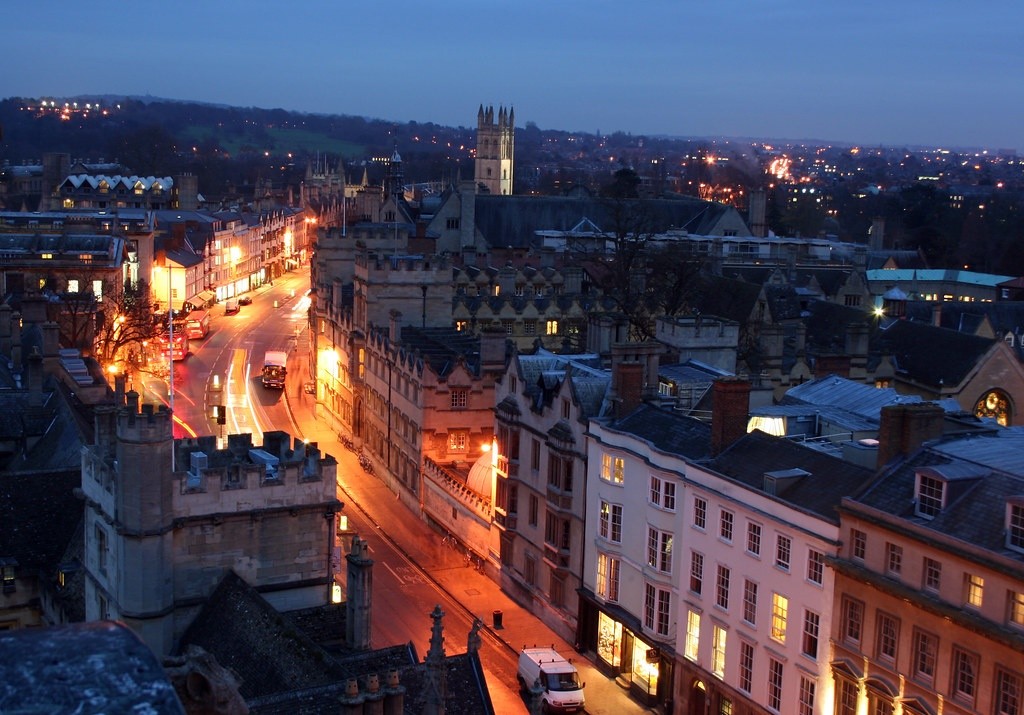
[225,299,241,315]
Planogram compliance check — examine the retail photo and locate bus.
[160,328,190,361]
[182,310,210,342]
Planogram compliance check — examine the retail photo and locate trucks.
[516,646,590,714]
[261,348,290,393]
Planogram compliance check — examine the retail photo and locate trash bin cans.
[493,610,503,628]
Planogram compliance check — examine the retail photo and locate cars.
[239,297,253,308]
